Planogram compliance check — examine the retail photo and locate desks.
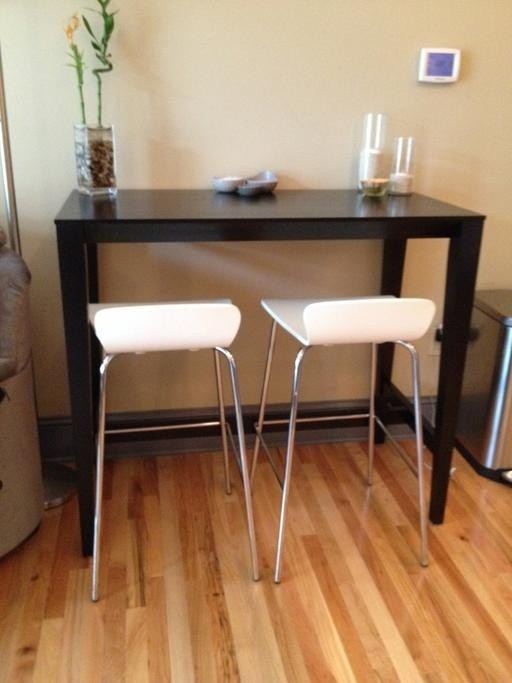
[53,188,487,558]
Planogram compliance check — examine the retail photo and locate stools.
[86,294,437,603]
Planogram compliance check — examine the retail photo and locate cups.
[355,111,388,193]
[386,135,418,197]
[359,178,390,197]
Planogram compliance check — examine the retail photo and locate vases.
[71,124,119,198]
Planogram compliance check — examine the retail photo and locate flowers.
[62,0,120,125]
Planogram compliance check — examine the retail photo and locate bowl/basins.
[245,169,278,193]
[212,175,245,194]
[237,183,263,197]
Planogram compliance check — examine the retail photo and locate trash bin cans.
[0,246,43,557]
[455,289,511,479]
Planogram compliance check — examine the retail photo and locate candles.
[359,149,381,187]
[391,172,412,195]
